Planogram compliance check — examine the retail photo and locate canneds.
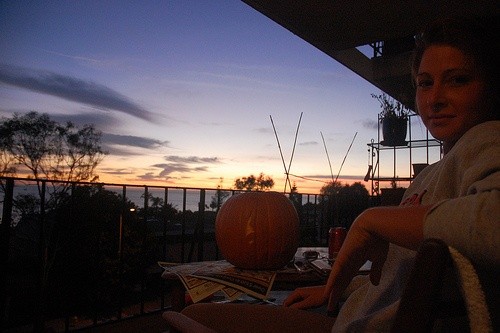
[328,227,346,265]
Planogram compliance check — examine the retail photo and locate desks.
[172,273,316,333]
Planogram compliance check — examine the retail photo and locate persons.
[181,17,500,333]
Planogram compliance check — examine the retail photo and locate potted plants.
[370,88,414,146]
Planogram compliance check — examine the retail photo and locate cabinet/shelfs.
[367,113,443,207]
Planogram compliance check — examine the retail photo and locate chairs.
[161,246,493,333]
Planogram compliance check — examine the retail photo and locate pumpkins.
[214,190,299,270]
